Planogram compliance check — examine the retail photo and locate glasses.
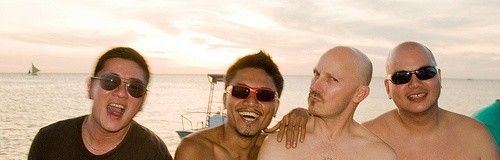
[91,74,148,98]
[388,65,437,85]
[225,85,278,102]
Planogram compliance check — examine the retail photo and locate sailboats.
[31,64,40,75]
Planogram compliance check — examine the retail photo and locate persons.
[364,42,500,160]
[28,47,174,160]
[256,46,398,160]
[174,50,309,160]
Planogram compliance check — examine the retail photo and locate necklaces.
[83,117,122,151]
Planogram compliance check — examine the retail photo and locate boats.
[174,73,228,138]
